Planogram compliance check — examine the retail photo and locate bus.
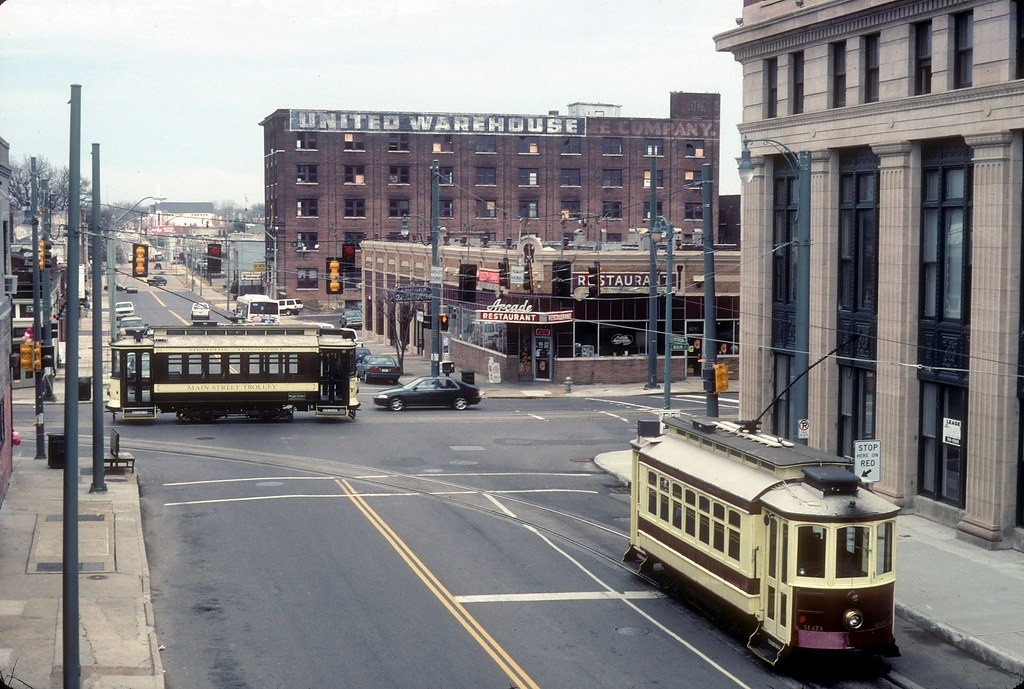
[232,293,279,324]
[622,413,901,671]
[107,324,362,424]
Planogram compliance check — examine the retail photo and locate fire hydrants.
[564,377,574,393]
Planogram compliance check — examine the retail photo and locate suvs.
[278,298,303,316]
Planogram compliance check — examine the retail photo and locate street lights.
[652,216,675,415]
[109,195,167,321]
[401,211,440,377]
[738,135,812,445]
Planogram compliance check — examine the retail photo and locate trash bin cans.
[46,433,64,468]
[79,376,93,401]
[459,370,476,385]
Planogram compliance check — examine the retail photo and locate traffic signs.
[853,440,882,482]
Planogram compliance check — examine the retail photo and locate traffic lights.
[207,244,221,273]
[341,244,356,273]
[39,240,52,269]
[439,315,448,332]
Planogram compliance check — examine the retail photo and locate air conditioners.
[3,274,18,296]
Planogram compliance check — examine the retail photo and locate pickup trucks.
[146,275,167,286]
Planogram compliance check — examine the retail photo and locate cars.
[353,347,403,385]
[373,376,482,412]
[115,302,136,320]
[117,316,146,336]
[99,251,225,319]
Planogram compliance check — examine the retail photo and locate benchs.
[104,429,135,473]
